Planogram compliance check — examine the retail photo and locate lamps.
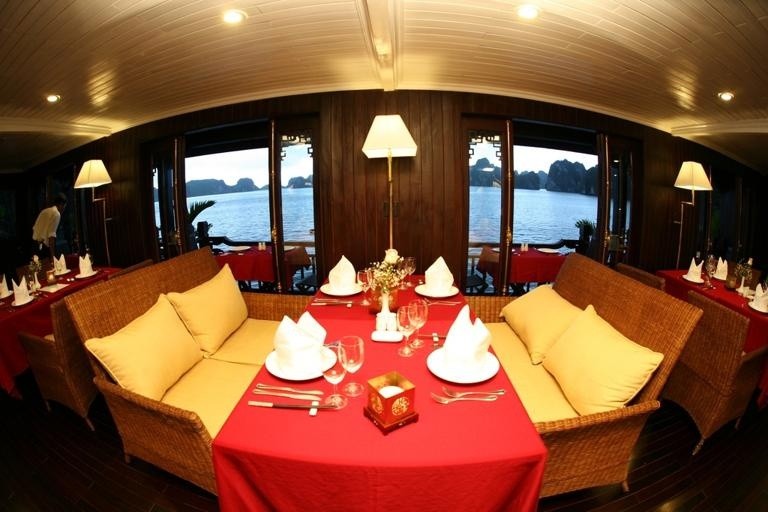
[73,159,113,267]
[674,162,713,271]
[362,114,417,285]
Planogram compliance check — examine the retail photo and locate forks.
[430,386,505,405]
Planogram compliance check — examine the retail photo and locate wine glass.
[321,257,428,410]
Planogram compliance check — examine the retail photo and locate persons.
[32,193,68,266]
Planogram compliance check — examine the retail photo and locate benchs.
[461,253,703,500]
[63,246,315,497]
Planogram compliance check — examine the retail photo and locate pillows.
[538,304,665,416]
[499,284,582,364]
[84,294,203,404]
[167,263,248,358]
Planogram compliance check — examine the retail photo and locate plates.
[0,269,98,307]
[683,274,768,313]
[284,246,296,252]
[491,247,515,252]
[537,248,560,254]
[265,283,500,385]
[228,245,251,251]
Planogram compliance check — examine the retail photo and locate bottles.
[257,241,266,251]
[520,241,528,252]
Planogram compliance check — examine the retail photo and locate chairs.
[614,257,768,462]
[464,255,489,296]
[0,254,153,432]
[295,251,317,292]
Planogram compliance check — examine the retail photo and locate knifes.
[247,383,339,410]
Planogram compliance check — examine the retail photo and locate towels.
[274,310,327,373]
[328,255,355,292]
[440,303,492,377]
[424,256,454,293]
